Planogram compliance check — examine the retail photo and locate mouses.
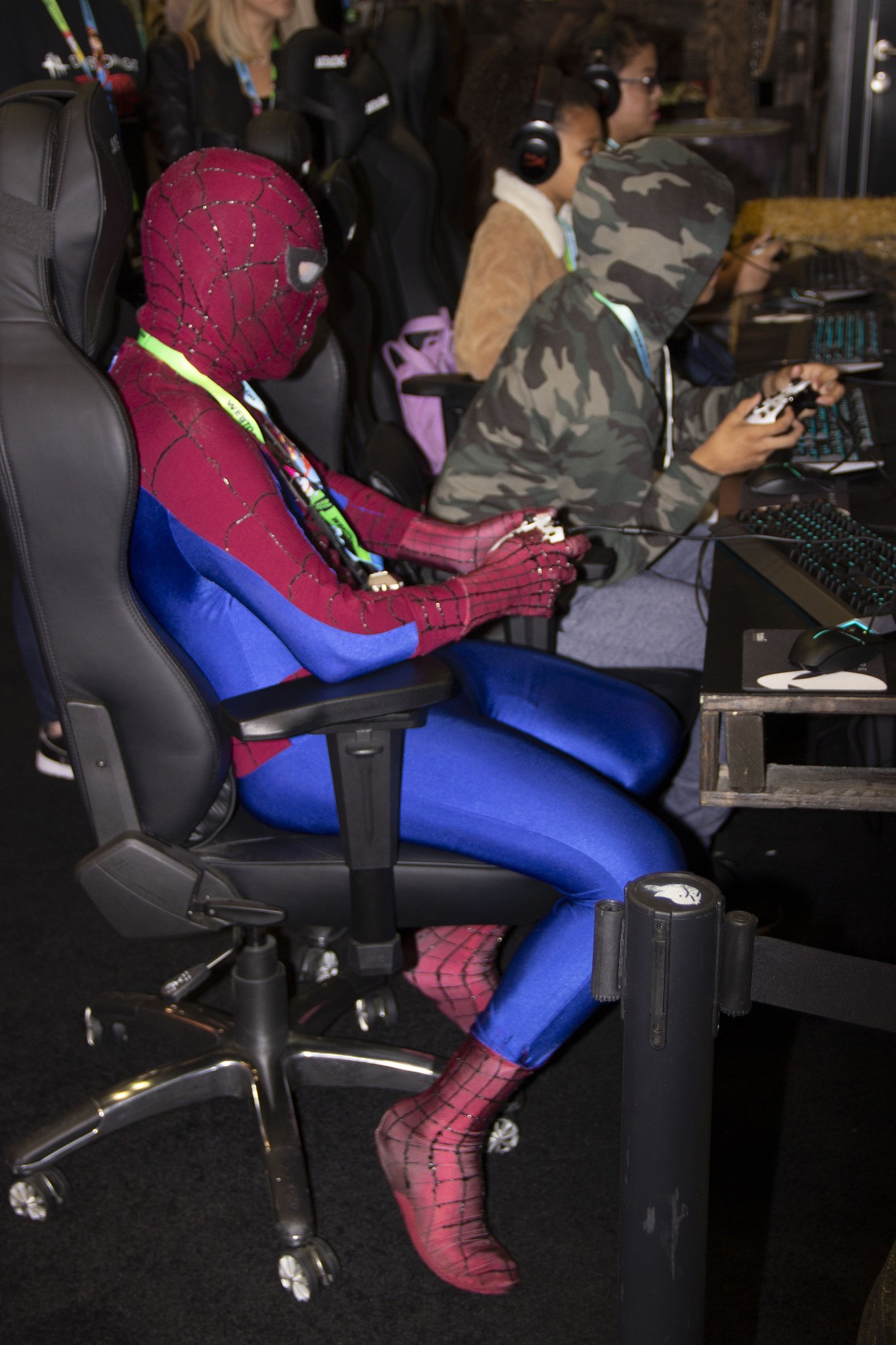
[788,625,880,674]
[745,464,835,498]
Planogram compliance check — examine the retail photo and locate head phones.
[508,56,562,187]
[579,16,623,121]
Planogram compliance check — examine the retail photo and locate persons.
[454,9,664,378]
[140,2,322,169]
[108,149,685,1292]
[427,135,847,848]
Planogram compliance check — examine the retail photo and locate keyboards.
[781,387,887,476]
[797,250,872,303]
[709,497,895,636]
[811,308,887,377]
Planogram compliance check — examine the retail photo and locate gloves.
[398,508,558,576]
[447,532,591,640]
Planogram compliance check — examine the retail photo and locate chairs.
[1,0,569,1301]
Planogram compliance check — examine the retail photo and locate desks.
[700,188,895,815]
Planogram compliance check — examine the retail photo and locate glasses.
[613,74,657,95]
